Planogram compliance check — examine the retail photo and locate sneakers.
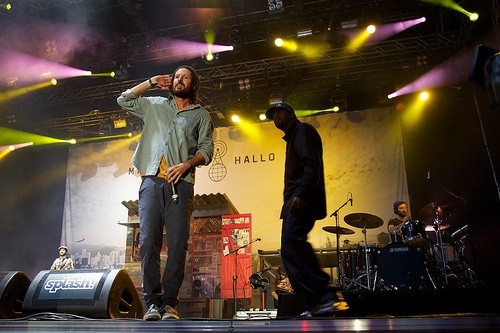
[143,303,162,321]
[160,304,180,321]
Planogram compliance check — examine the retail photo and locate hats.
[393,200,406,214]
[58,245,69,252]
[265,103,294,120]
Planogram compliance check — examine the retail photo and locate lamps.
[239,78,255,91]
[269,97,282,104]
[341,20,358,29]
[113,119,127,128]
[268,0,284,15]
[297,28,312,37]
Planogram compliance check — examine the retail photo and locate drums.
[358,242,381,273]
[375,241,432,291]
[388,230,402,243]
[427,243,456,265]
[400,219,428,244]
[338,245,362,280]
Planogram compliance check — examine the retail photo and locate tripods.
[345,222,385,291]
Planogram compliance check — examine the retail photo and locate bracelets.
[148,78,154,88]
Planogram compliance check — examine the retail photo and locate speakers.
[0,268,145,322]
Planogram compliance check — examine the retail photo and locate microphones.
[169,176,179,204]
[427,171,430,187]
[257,238,261,241]
[351,193,353,206]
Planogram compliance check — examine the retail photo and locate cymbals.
[418,199,457,225]
[343,213,384,229]
[425,224,450,232]
[322,226,356,235]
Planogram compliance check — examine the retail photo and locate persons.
[116,66,214,319]
[50,246,74,270]
[265,103,339,319]
[388,200,422,244]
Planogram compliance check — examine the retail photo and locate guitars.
[249,273,293,294]
[57,261,71,270]
